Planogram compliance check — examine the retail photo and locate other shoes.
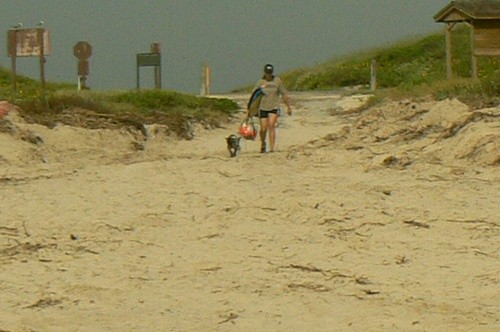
[259,143,267,153]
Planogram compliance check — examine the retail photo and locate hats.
[263,63,274,73]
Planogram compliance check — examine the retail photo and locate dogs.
[224,134,242,158]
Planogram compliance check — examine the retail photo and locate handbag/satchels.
[240,115,257,141]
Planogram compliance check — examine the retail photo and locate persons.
[246,64,292,152]
